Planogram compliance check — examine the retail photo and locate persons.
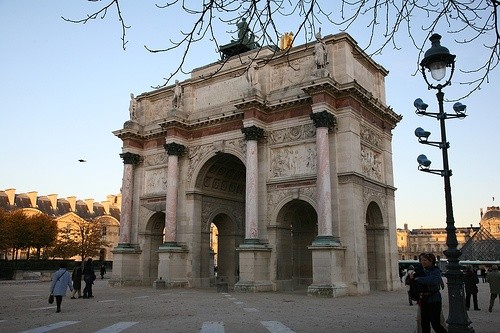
[236,18,250,39]
[399,253,500,333]
[70,262,83,299]
[100,264,106,280]
[81,258,96,299]
[49,262,73,313]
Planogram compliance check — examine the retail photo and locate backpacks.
[409,268,436,301]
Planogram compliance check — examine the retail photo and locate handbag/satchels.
[49,293,54,304]
[87,274,96,282]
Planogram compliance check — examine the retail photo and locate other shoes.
[473,308,480,311]
[57,308,60,313]
[78,296,83,298]
[489,307,492,312]
[71,296,76,299]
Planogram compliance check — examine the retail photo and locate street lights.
[414,34,480,333]
